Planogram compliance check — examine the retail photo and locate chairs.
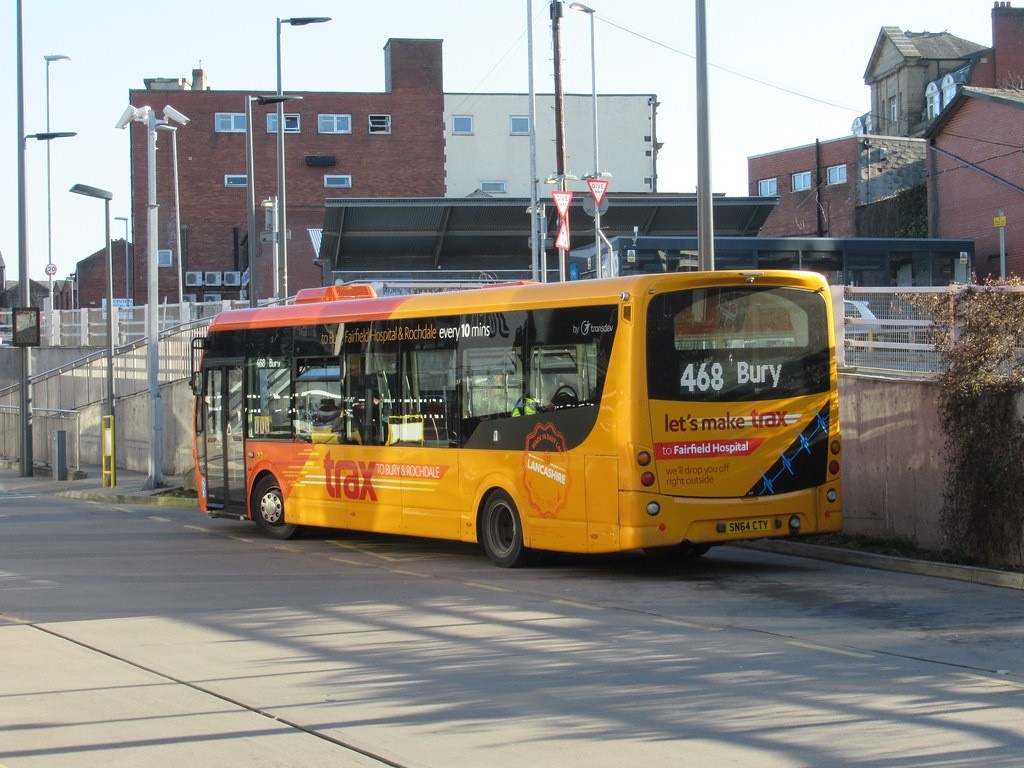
[546,386,579,411]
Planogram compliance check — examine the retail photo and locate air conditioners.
[205,271,222,286]
[185,271,202,286]
[203,294,221,308]
[183,294,196,302]
[224,271,240,287]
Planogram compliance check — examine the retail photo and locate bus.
[188,270,844,565]
[593,233,976,335]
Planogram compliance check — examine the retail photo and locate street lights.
[13,0,76,474]
[272,15,333,294]
[114,216,131,344]
[69,183,120,487]
[244,94,305,310]
[43,53,73,342]
[570,6,602,278]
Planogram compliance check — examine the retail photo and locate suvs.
[843,300,884,352]
